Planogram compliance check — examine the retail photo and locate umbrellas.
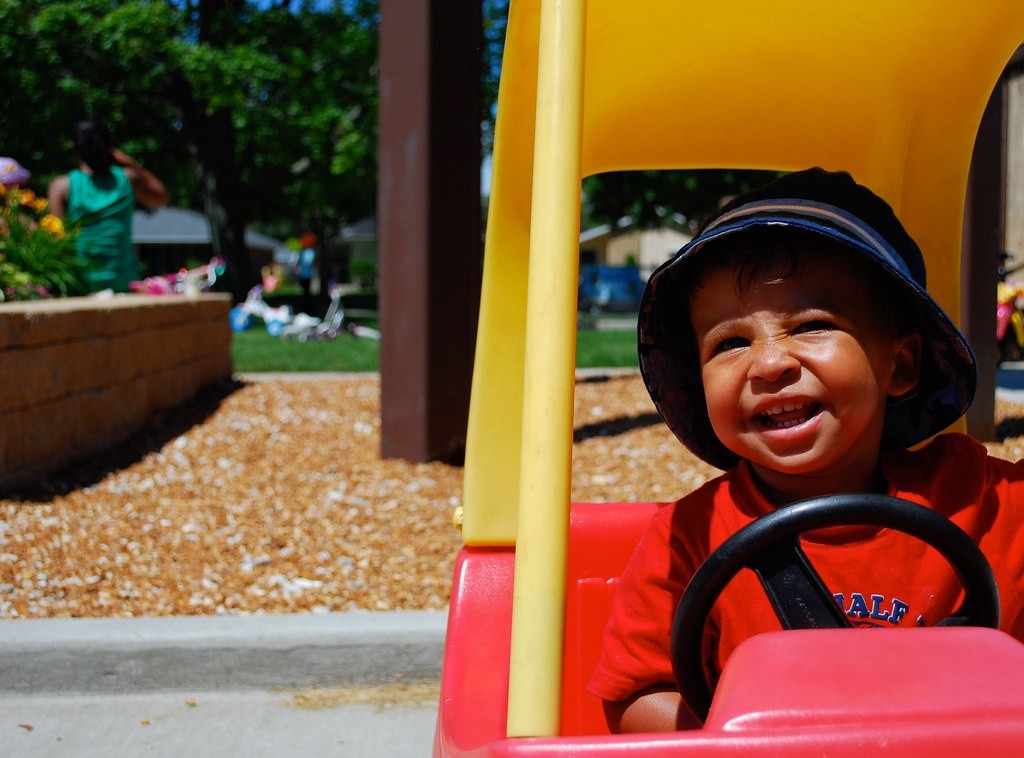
[130,204,286,248]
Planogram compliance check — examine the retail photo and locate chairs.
[431,499,691,758]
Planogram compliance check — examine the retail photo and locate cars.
[576,264,649,316]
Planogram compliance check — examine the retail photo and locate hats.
[637,167,978,471]
[0,157,30,184]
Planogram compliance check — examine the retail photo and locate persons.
[0,140,166,292]
[601,164,1024,732]
